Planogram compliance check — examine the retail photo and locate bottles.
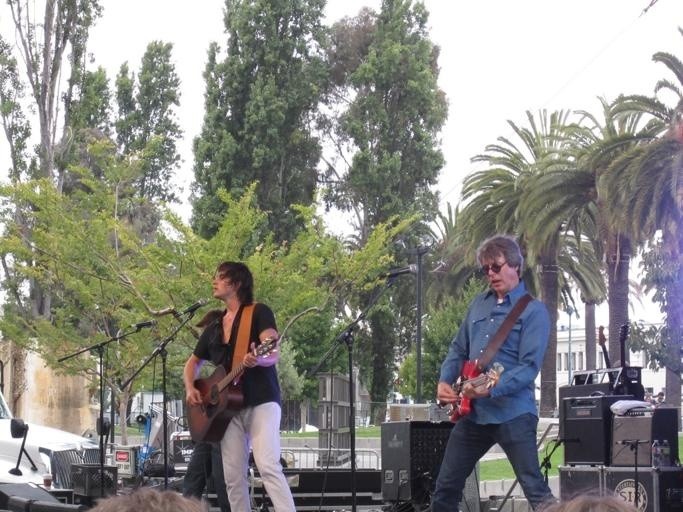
[651,438,671,468]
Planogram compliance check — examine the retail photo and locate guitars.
[614,324,644,401]
[186,334,278,442]
[445,359,504,423]
[598,326,615,383]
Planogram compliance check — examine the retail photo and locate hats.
[195,309,223,327]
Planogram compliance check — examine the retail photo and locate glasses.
[480,260,509,275]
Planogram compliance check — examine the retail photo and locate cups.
[43,474,52,490]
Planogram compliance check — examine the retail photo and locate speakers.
[611,407,678,469]
[562,394,634,468]
[380,422,455,502]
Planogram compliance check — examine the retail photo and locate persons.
[430,236,559,512]
[183,261,297,512]
[535,486,642,512]
[87,488,209,512]
[182,311,232,512]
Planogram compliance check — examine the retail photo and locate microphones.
[384,263,418,278]
[184,297,208,315]
[136,318,157,328]
